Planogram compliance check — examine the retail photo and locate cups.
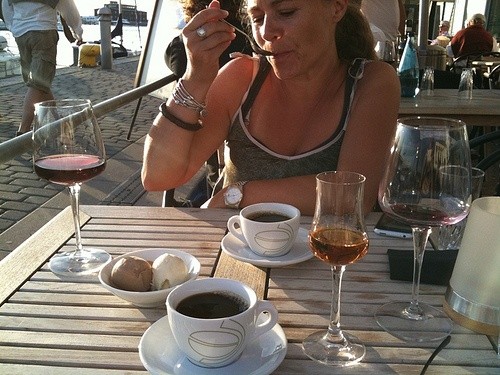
[377,40,395,63]
[437,165,485,251]
[442,195,500,338]
[228,202,301,258]
[165,276,279,369]
[420,66,436,97]
[457,68,474,100]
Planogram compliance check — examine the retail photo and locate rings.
[195,26,208,40]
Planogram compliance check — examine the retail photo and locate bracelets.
[158,102,200,131]
[171,78,207,113]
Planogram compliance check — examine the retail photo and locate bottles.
[396,19,420,98]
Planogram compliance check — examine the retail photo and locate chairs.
[449,51,500,89]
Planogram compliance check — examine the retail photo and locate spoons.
[220,18,275,56]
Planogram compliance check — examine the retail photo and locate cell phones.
[374,213,413,238]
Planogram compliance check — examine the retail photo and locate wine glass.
[30,99,113,278]
[300,171,370,368]
[373,116,473,344]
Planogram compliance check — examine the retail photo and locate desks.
[399,89,500,126]
[0,205,500,375]
[471,60,500,89]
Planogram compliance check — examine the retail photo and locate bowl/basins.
[98,247,202,308]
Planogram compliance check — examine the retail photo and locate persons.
[163,0,261,188]
[0,0,83,156]
[140,0,400,217]
[429,13,499,73]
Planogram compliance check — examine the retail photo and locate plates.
[221,227,314,268]
[138,311,289,375]
